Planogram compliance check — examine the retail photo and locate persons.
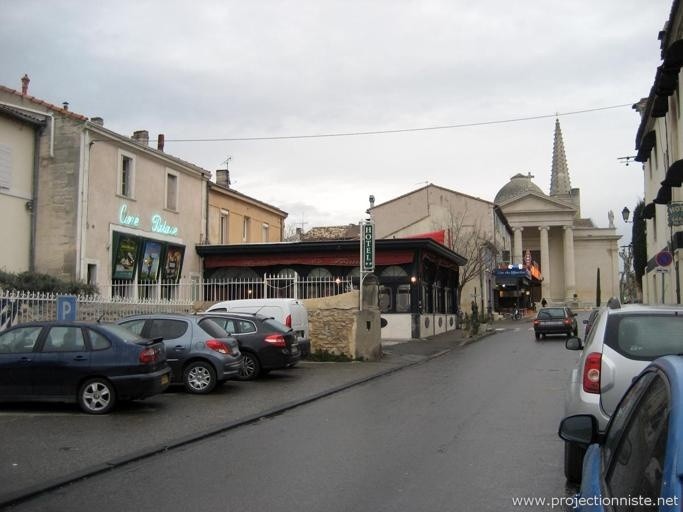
[541,298,547,307]
[470,301,478,318]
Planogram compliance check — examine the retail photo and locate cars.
[203,312,302,378]
[534,307,578,340]
[558,298,683,512]
[117,314,241,394]
[0,322,171,415]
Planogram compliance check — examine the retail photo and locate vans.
[205,298,311,366]
